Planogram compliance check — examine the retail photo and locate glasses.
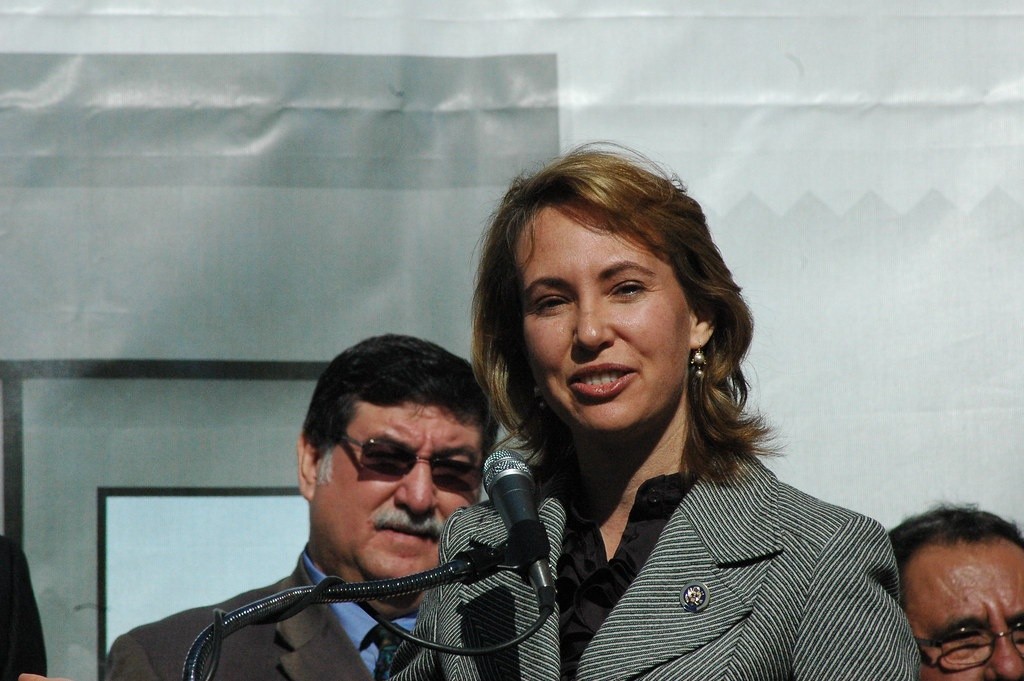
[915,622,1024,665]
[340,433,484,493]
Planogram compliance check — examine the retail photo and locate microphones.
[483,449,555,614]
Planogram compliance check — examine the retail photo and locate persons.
[0,531,46,680]
[103,334,500,681]
[890,505,1024,681]
[388,141,923,680]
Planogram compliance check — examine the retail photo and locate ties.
[369,622,409,681]
